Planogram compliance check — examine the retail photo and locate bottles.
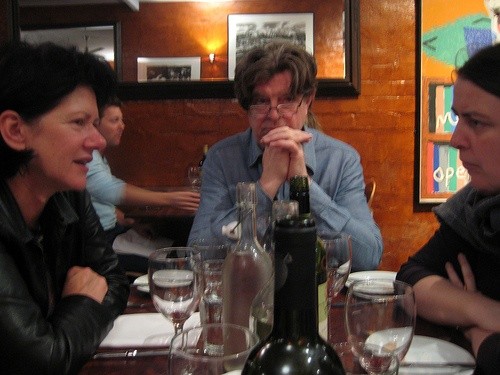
[241,217,346,375]
[274,175,328,342]
[220,182,275,371]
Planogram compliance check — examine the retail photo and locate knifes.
[93,349,170,359]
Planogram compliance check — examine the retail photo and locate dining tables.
[84,260,481,375]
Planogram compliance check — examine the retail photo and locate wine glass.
[148,246,205,335]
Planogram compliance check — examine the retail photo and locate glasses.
[249,93,309,114]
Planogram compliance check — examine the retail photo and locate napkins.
[99,310,206,349]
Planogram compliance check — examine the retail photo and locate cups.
[189,237,231,357]
[168,322,260,375]
[319,232,352,313]
[344,278,416,375]
[334,343,399,375]
[187,165,202,191]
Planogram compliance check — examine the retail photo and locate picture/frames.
[137,56,201,83]
[413,0,500,211]
[228,13,313,80]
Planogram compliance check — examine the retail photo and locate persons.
[81,91,200,276]
[0,38,131,375]
[394,40,499,375]
[187,42,385,275]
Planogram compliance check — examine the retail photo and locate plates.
[345,271,399,303]
[134,269,193,292]
[359,328,476,375]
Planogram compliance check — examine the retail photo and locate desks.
[124,185,199,245]
